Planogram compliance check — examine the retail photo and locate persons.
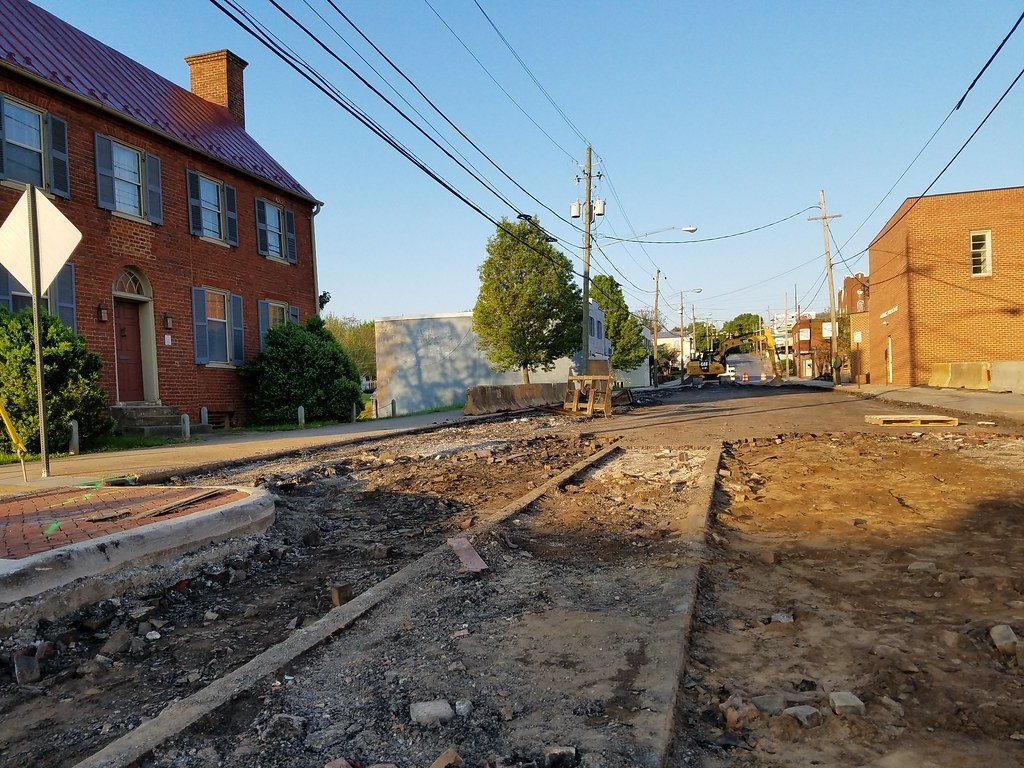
[833,352,842,387]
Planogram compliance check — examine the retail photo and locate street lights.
[653,287,703,389]
[580,226,699,403]
[691,311,713,360]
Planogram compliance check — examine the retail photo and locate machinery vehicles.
[685,326,785,388]
